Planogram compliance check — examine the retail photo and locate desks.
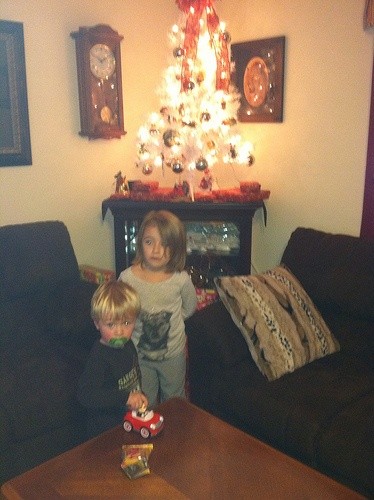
[0,398,368,500]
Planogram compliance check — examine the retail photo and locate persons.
[117,211,198,409]
[78,280,148,441]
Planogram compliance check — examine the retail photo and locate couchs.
[186,227,374,500]
[0,221,100,486]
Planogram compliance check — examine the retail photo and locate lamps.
[133,0,256,172]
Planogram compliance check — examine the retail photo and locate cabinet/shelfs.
[103,199,264,289]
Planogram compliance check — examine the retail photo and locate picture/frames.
[0,20,32,167]
[230,36,285,123]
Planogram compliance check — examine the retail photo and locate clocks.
[70,24,127,140]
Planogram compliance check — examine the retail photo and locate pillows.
[214,262,340,382]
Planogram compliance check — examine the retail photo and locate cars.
[123,408,166,439]
